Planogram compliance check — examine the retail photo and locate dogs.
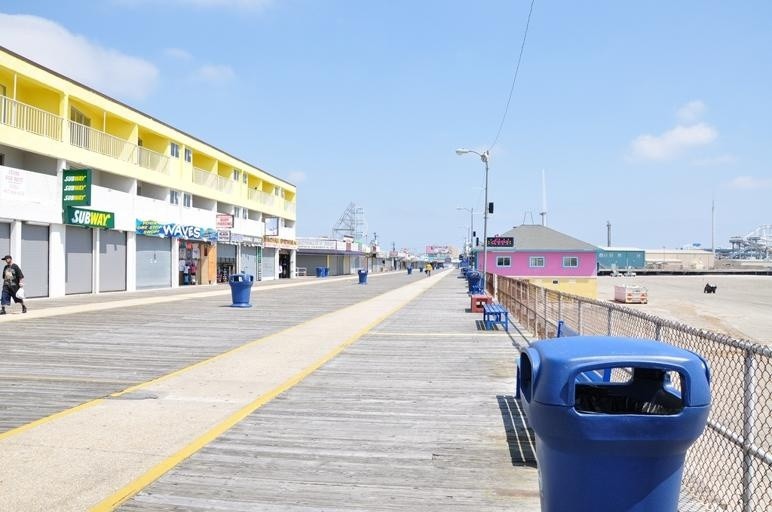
[704,283,717,293]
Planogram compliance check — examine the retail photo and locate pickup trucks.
[610,271,636,277]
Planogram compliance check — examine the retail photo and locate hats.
[2,256,12,260]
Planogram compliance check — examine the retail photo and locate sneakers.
[22,307,26,313]
[0,310,6,314]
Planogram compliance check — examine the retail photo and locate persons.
[424,262,432,277]
[0,254,27,316]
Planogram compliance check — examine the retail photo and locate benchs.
[557,320,612,384]
[483,304,508,330]
[471,295,492,313]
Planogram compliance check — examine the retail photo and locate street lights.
[455,148,489,293]
[456,207,474,269]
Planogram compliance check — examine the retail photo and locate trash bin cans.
[516,335,712,512]
[229,275,253,307]
[358,270,368,283]
[316,268,328,277]
[407,266,412,274]
[419,267,423,272]
[467,271,480,293]
